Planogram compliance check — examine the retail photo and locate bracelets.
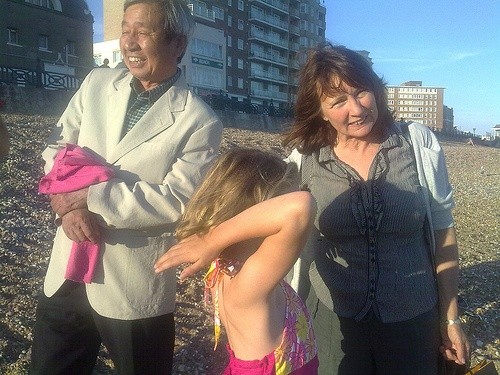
[440,317,462,328]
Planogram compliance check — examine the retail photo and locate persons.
[29,0,224,375]
[152,148,320,374]
[278,46,471,375]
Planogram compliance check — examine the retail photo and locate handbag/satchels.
[441,347,499,374]
